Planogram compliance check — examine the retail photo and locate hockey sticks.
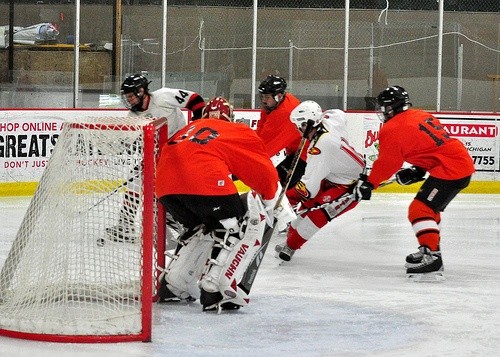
[293,178,397,215]
[236,139,305,295]
[80,172,143,215]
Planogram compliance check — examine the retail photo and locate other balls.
[97,238,105,246]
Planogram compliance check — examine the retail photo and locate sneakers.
[275,243,286,258]
[279,245,295,265]
[405,245,423,267]
[406,245,445,281]
[200,288,241,313]
[158,282,196,303]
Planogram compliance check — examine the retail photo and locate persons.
[354,84,476,282]
[274,100,367,263]
[367,55,388,110]
[212,55,236,100]
[253,75,309,190]
[155,94,281,313]
[106,73,203,244]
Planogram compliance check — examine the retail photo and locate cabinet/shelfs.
[0,0,121,94]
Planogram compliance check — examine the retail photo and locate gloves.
[349,172,374,201]
[395,164,426,185]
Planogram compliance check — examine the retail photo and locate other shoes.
[105,226,136,243]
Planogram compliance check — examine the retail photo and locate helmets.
[120,73,152,112]
[290,99,323,128]
[375,85,412,122]
[258,74,287,103]
[203,96,235,121]
